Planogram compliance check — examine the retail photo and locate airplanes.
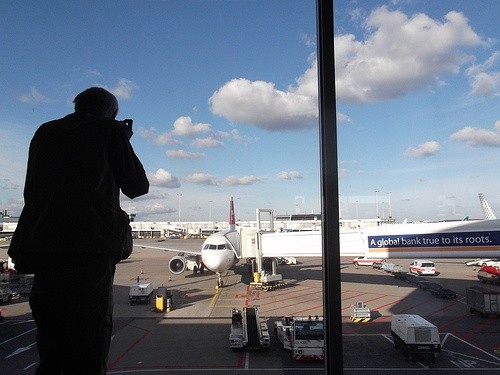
[133,196,241,288]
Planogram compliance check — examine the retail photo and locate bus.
[132,229,168,239]
[201,229,227,238]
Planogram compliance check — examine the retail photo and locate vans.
[409,259,436,276]
[353,256,384,268]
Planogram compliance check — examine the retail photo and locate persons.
[8,86,150,375]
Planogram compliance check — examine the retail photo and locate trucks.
[274,314,324,362]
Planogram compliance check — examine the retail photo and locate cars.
[465,258,500,285]
